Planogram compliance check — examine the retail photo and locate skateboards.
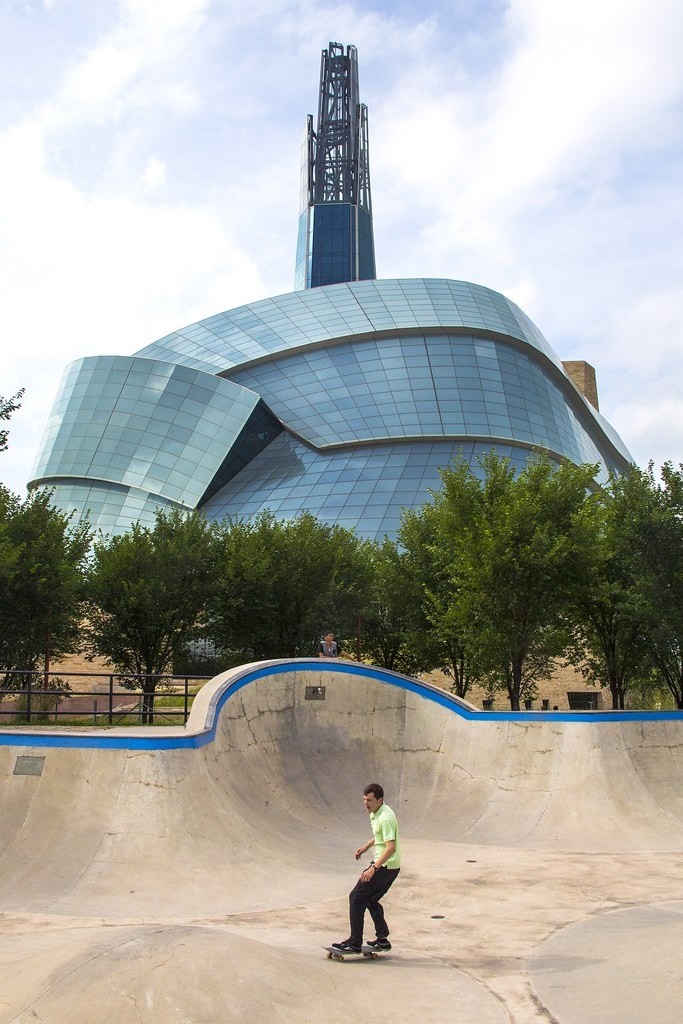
[321,945,383,962]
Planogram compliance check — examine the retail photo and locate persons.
[317,630,356,662]
[333,783,401,956]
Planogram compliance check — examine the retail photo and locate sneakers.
[331,937,362,954]
[366,938,392,952]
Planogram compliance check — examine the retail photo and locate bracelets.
[372,865,378,871]
